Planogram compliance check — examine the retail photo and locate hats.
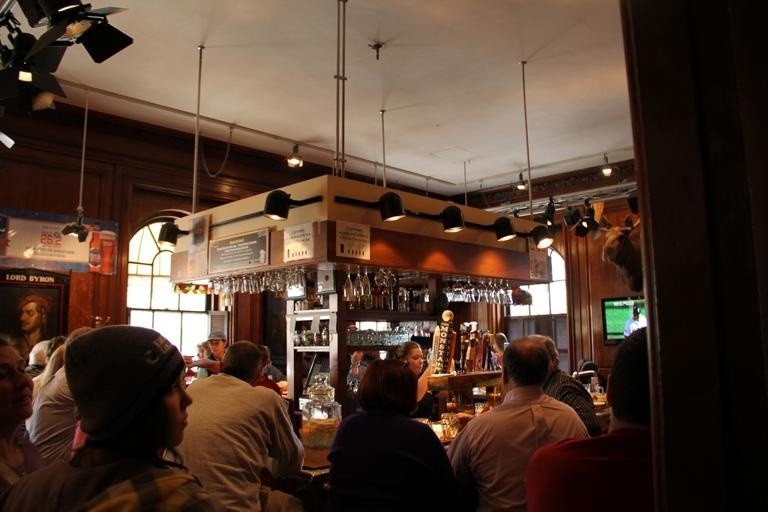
[207,330,225,342]
[64,324,186,433]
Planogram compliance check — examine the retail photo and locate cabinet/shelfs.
[285,263,444,420]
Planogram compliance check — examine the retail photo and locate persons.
[398,342,442,422]
[177,341,305,511]
[0,327,227,511]
[13,294,51,358]
[328,361,453,509]
[624,304,648,336]
[492,333,509,365]
[525,324,650,511]
[251,345,281,396]
[451,340,588,509]
[528,334,597,435]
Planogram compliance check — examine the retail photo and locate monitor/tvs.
[601,295,647,346]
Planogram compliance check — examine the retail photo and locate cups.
[99,230,118,274]
[474,403,485,415]
[441,414,460,438]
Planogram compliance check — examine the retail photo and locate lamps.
[488,217,516,241]
[600,155,612,177]
[158,223,188,247]
[263,190,304,221]
[529,225,554,249]
[515,173,527,189]
[434,206,464,233]
[372,191,406,222]
[61,214,89,242]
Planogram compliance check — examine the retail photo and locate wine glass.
[183,355,196,377]
[445,275,514,304]
[206,265,305,294]
[344,263,396,298]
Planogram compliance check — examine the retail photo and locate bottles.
[346,326,415,346]
[294,326,329,346]
[88,219,103,274]
[348,285,429,313]
